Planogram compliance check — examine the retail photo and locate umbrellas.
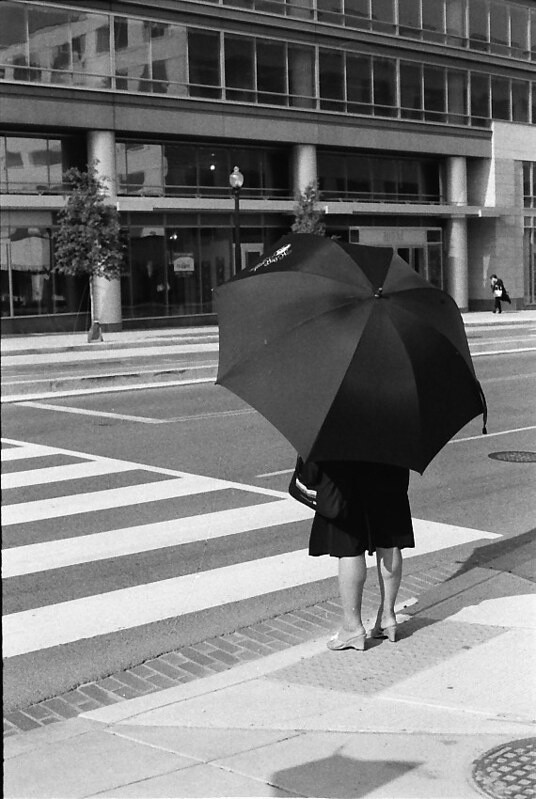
[211,233,487,476]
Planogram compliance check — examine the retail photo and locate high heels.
[369,614,397,642]
[327,621,366,651]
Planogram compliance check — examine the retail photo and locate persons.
[490,275,505,313]
[309,461,415,651]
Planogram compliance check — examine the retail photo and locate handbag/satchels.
[288,453,322,511]
[493,286,503,298]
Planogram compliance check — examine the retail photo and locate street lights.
[229,166,245,278]
